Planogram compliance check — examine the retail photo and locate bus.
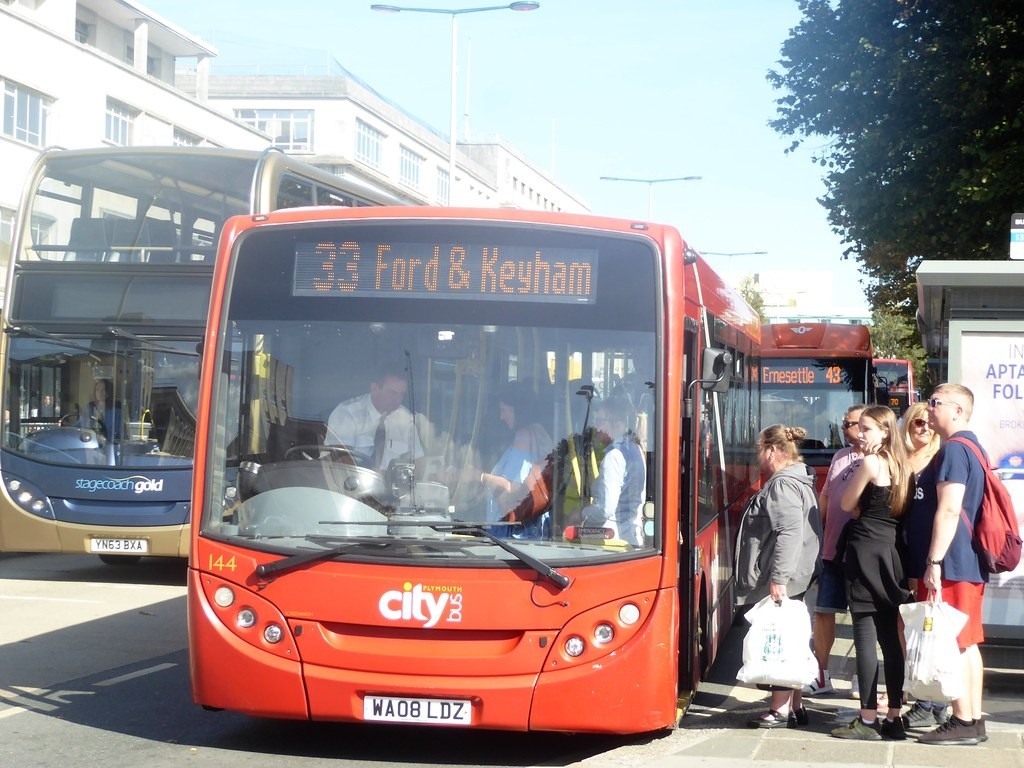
[0,143,523,568]
[187,203,764,738]
[873,358,923,420]
[760,322,891,498]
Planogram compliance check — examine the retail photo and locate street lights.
[597,175,701,222]
[369,0,542,207]
[575,383,595,497]
[699,251,770,286]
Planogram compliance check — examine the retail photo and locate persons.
[733,424,824,728]
[38,393,53,418]
[317,344,655,548]
[23,384,33,417]
[802,382,991,747]
[71,379,122,441]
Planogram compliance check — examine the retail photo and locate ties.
[371,417,386,472]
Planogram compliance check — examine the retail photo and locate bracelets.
[926,557,942,566]
[579,505,588,521]
[480,473,485,485]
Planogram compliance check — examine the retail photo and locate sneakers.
[917,715,979,746]
[879,716,906,740]
[831,714,884,741]
[974,718,988,742]
[933,704,951,724]
[847,674,860,699]
[801,668,832,697]
[900,703,932,728]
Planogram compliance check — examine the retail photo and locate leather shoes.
[794,707,809,725]
[746,710,797,729]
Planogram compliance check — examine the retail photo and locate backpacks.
[938,437,1023,573]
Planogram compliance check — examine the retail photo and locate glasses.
[842,420,860,429]
[927,398,958,408]
[912,418,935,429]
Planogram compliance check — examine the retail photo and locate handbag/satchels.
[504,429,556,524]
[899,583,969,703]
[735,591,820,690]
